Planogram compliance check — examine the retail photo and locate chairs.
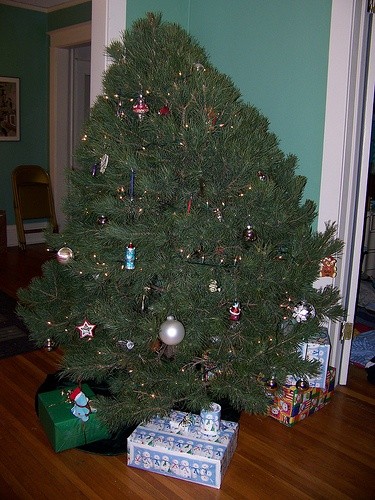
[11,164,59,254]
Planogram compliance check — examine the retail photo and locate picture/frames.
[0,75,22,143]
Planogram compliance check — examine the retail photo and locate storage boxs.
[126,409,240,490]
[267,327,336,427]
[39,384,110,454]
